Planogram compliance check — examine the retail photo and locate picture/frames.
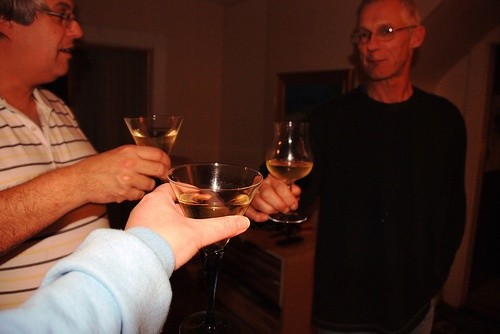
[273,68,355,123]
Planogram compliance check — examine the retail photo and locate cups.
[124,117,184,182]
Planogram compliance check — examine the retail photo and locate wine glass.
[265,121,314,223]
[167,164,263,334]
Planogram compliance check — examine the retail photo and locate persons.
[243,0,467,334]
[0,181,250,334]
[0,0,173,311]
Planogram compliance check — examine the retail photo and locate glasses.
[350,23,418,44]
[35,9,76,28]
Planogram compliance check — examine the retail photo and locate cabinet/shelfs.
[209,226,317,334]
